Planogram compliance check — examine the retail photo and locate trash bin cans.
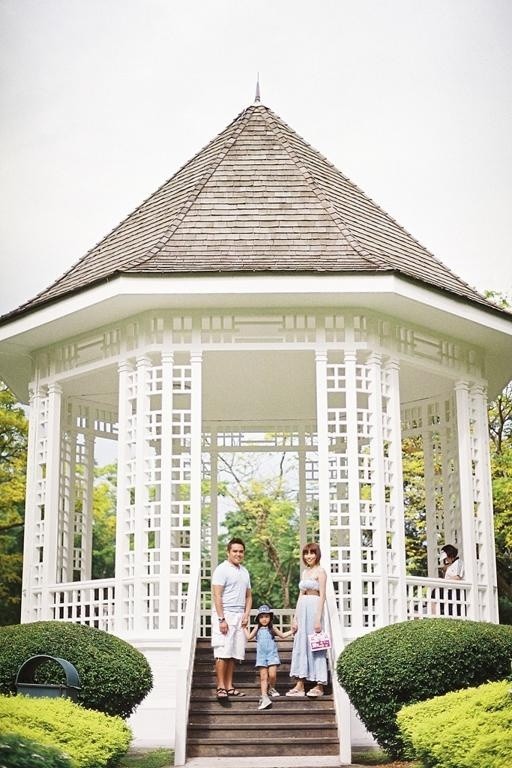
[15,655,81,703]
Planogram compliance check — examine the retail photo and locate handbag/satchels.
[308,630,331,654]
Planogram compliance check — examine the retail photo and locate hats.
[439,549,448,562]
[254,604,275,622]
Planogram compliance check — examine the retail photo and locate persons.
[285,543,345,697]
[211,539,253,699]
[242,605,298,710]
[442,545,464,616]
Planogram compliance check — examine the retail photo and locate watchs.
[218,617,225,623]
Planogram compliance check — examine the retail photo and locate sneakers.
[286,688,307,697]
[267,687,281,698]
[258,695,271,711]
[307,687,325,697]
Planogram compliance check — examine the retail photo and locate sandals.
[216,687,228,699]
[226,689,247,697]
